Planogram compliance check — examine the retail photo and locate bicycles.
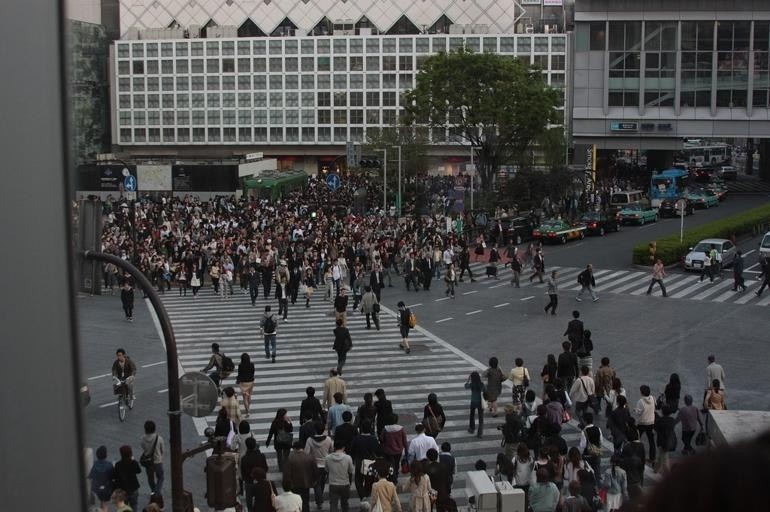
[111,376,134,422]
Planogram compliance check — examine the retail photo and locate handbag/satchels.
[372,498,383,512]
[587,443,600,455]
[373,304,380,313]
[656,397,663,410]
[589,394,599,408]
[696,432,705,446]
[401,458,410,475]
[421,414,442,434]
[480,396,489,410]
[140,456,155,467]
[276,434,295,446]
[524,375,529,386]
[409,314,417,327]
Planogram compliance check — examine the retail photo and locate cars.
[575,210,622,236]
[616,201,660,226]
[716,166,737,179]
[759,232,770,259]
[533,220,587,244]
[687,188,725,210]
[661,196,695,218]
[684,239,741,271]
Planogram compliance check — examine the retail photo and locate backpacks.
[264,314,274,332]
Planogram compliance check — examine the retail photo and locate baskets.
[113,382,128,396]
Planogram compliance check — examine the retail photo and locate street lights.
[392,145,402,218]
[470,146,482,210]
[374,149,387,212]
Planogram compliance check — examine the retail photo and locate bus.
[243,169,308,206]
[650,168,690,210]
[672,145,732,169]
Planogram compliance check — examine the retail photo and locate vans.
[610,190,644,208]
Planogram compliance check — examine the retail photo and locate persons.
[69,139,770,512]
